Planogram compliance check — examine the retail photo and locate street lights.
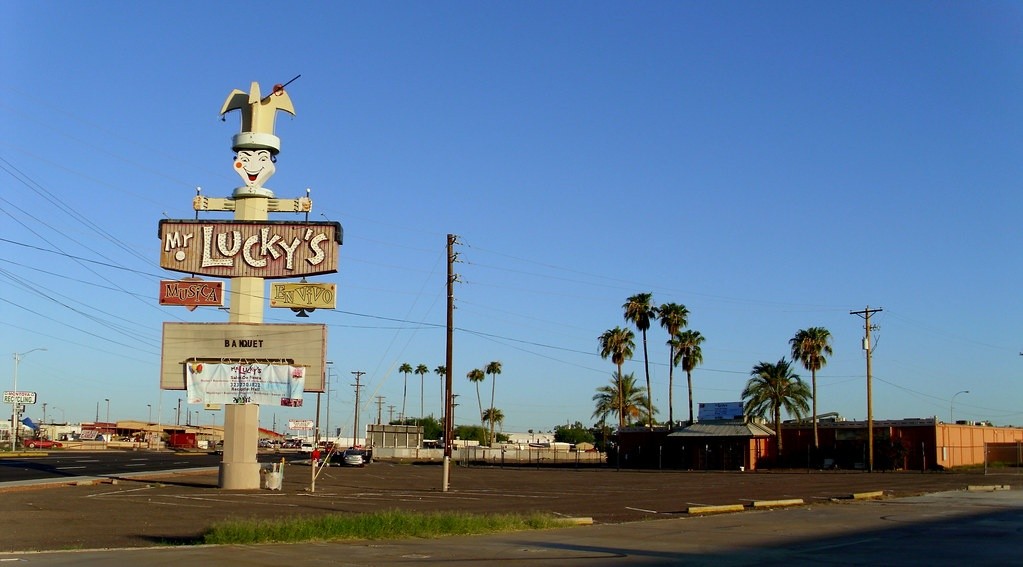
[195,410,199,445]
[10,346,49,453]
[52,406,66,423]
[173,407,178,449]
[147,403,151,448]
[211,413,216,449]
[104,398,110,450]
[42,401,48,424]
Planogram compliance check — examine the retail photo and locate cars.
[339,449,365,467]
[23,436,64,449]
[258,437,314,451]
